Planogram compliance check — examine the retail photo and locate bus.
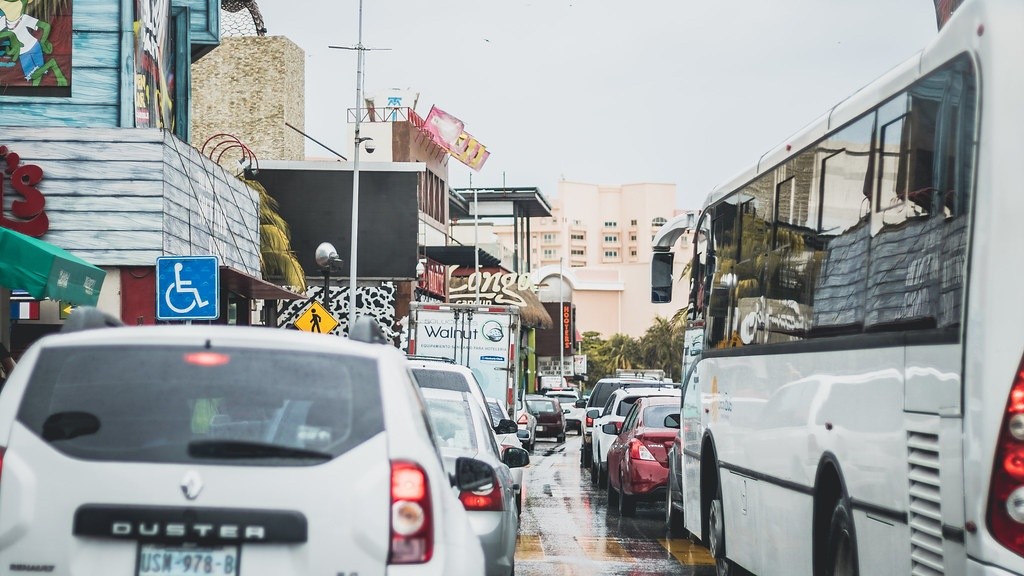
[647,0,1024,576]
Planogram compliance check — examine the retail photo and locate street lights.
[348,43,376,337]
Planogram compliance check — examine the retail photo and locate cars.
[0,308,497,576]
[261,353,531,576]
[418,368,705,546]
